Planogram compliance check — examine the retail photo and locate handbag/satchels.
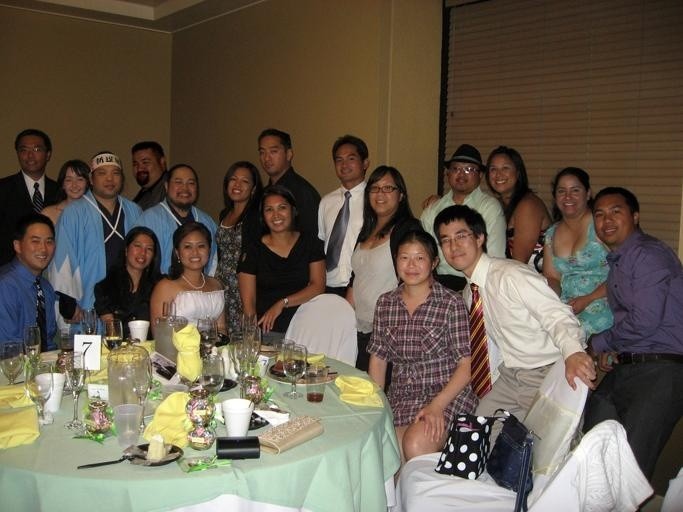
[487,408,541,512]
[434,411,498,481]
[257,415,324,456]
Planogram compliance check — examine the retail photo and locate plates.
[122,443,183,466]
[265,360,341,386]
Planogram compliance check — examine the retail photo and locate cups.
[103,319,124,352]
[305,366,327,402]
[79,312,98,335]
[222,398,254,437]
[271,338,294,365]
[24,326,42,362]
[112,404,145,446]
[2,339,24,384]
[128,320,151,343]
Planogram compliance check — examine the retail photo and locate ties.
[469,284,492,399]
[326,192,352,272]
[33,183,44,214]
[35,278,48,351]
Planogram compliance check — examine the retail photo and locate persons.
[366,230,479,489]
[1,129,226,357]
[317,135,370,298]
[419,144,507,283]
[422,145,553,273]
[542,168,614,398]
[346,166,423,393]
[215,129,325,347]
[582,188,683,483]
[434,205,596,455]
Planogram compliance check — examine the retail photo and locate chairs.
[526,419,620,512]
[284,293,359,368]
[398,357,589,511]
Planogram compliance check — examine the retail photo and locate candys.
[55,351,72,374]
[83,401,110,431]
[240,375,265,401]
[186,389,216,450]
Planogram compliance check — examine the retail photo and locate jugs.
[154,314,188,365]
[107,346,154,434]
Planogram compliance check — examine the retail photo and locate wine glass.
[283,342,307,400]
[65,351,85,432]
[25,361,55,425]
[196,311,268,402]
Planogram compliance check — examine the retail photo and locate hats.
[442,144,485,170]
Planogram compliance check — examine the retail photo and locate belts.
[616,351,681,365]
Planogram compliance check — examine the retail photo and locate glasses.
[367,184,397,193]
[19,145,44,153]
[449,166,476,174]
[442,233,470,248]
